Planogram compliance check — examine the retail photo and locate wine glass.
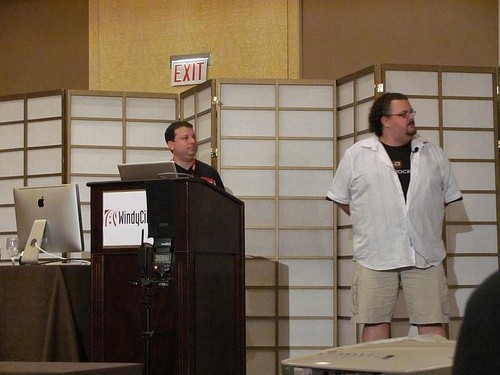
[6,238,20,266]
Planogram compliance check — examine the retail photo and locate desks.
[281,333,457,375]
[0,266,92,362]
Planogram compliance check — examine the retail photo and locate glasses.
[383,111,416,118]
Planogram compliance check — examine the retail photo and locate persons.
[156,121,225,191]
[325,91,464,345]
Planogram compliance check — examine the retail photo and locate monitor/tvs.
[13,184,85,265]
[118,161,178,181]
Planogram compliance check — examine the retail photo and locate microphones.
[415,147,419,152]
